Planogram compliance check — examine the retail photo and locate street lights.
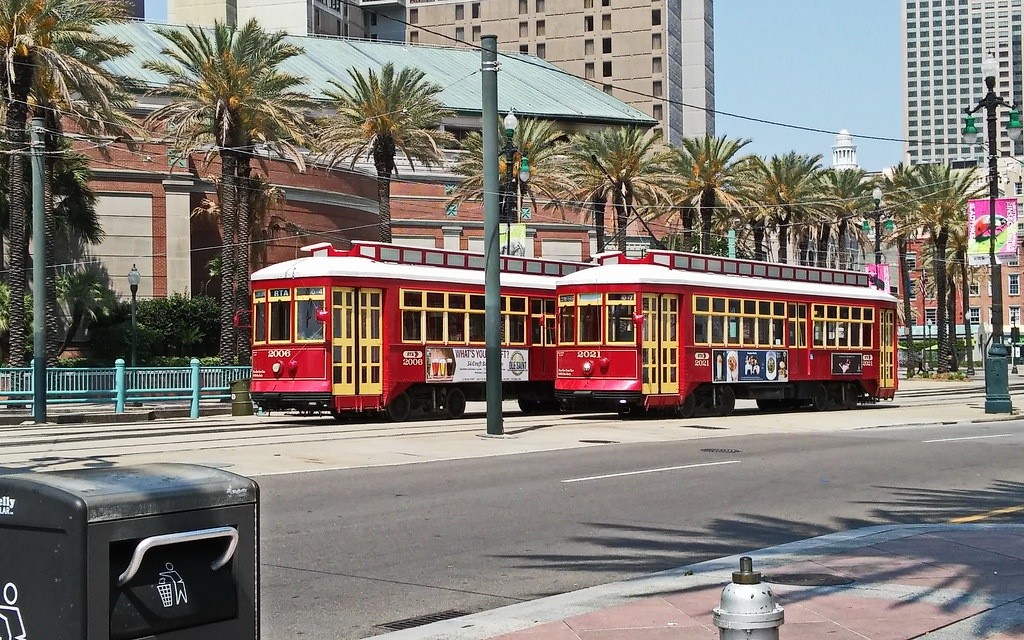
[960,51,1024,414]
[928,319,933,371]
[128,263,141,365]
[1009,307,1019,374]
[499,112,530,253]
[863,187,895,289]
[964,310,974,375]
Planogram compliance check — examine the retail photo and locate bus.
[234,241,602,425]
[554,247,900,421]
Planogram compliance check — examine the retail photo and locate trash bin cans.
[230,377,253,416]
[0,460,261,640]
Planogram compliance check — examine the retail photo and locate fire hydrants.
[711,555,785,640]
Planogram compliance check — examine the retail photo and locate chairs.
[450,329,462,341]
